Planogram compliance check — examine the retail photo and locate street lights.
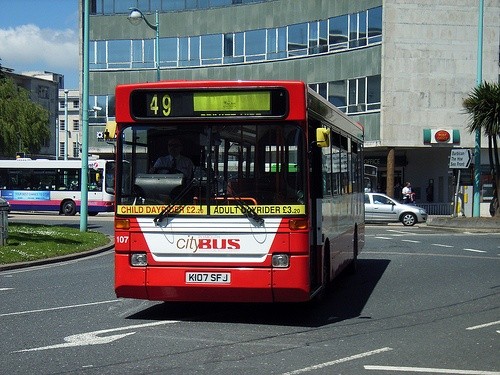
[126,6,161,81]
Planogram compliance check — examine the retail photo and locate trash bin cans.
[0,198,12,246]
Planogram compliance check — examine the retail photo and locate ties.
[172,159,177,170]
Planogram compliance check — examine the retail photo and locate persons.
[69,175,79,190]
[364,183,372,192]
[402,181,416,205]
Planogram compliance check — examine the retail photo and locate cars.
[364,192,428,226]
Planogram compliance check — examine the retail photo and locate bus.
[0,154,131,217]
[102,79,366,304]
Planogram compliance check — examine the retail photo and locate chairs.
[227,167,355,197]
